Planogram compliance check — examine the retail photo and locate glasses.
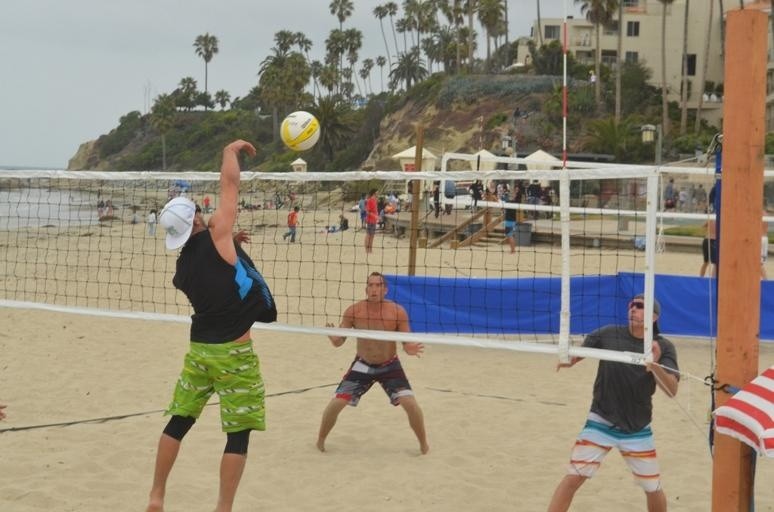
[195,205,202,214]
[629,302,644,309]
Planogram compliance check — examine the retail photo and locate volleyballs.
[280,109,321,152]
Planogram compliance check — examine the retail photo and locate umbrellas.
[711,365,774,457]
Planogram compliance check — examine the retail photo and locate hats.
[633,294,661,335]
[160,198,196,249]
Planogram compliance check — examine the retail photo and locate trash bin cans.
[467,223,482,238]
[515,223,532,247]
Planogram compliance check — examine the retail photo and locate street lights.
[642,123,663,168]
[501,135,517,196]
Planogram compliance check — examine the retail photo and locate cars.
[428,182,476,211]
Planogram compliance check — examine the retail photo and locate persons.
[147,139,276,512]
[432,181,558,253]
[329,215,349,232]
[547,294,680,512]
[664,180,707,213]
[283,207,299,243]
[701,187,717,276]
[358,189,399,252]
[98,195,209,237]
[316,272,429,455]
